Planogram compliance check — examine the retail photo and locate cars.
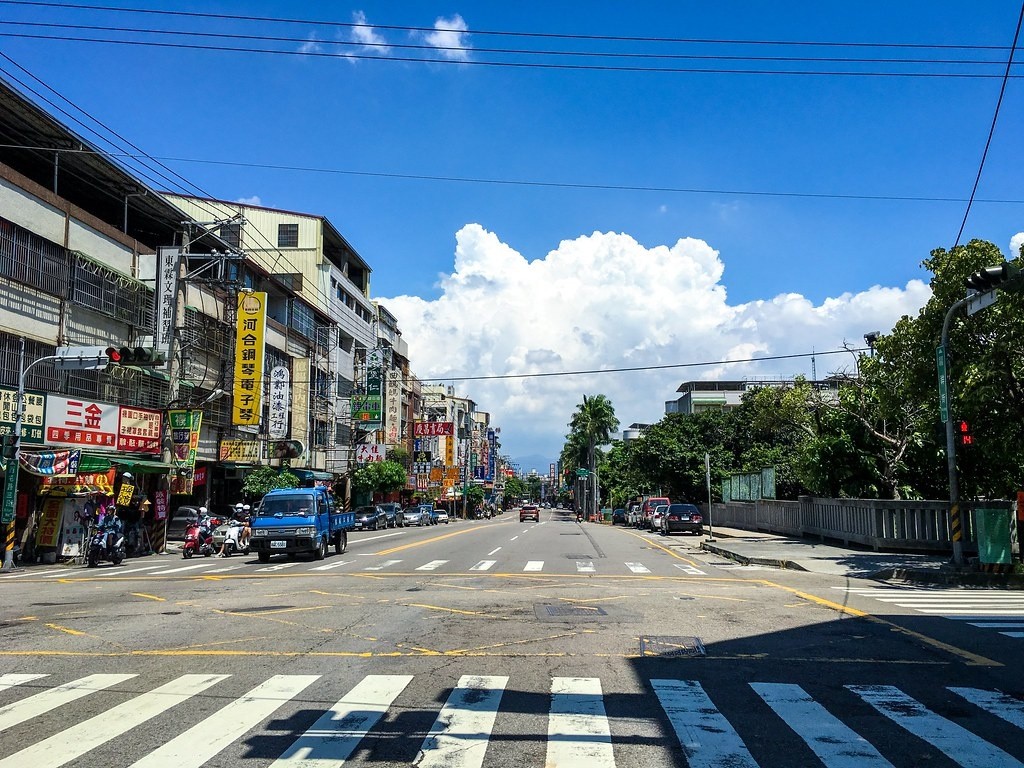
[212,515,248,554]
[434,509,449,524]
[402,506,431,526]
[518,504,540,522]
[353,504,389,531]
[659,503,704,535]
[649,505,669,531]
[165,504,228,542]
[556,502,563,509]
[610,508,628,526]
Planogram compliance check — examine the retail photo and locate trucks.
[245,482,355,562]
[416,503,440,526]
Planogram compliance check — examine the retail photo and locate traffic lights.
[957,418,973,446]
[964,260,1011,292]
[105,346,155,365]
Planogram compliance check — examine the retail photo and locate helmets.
[105,505,116,516]
[253,508,258,514]
[235,503,243,513]
[197,507,207,516]
[243,505,250,515]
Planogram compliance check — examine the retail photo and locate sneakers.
[200,543,208,548]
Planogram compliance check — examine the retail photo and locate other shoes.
[108,552,112,556]
[239,541,244,546]
[246,542,249,546]
[219,553,225,557]
[214,553,222,558]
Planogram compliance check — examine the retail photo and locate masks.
[245,511,249,513]
[237,509,242,512]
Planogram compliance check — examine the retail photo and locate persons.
[195,503,252,556]
[98,505,122,556]
[68,499,81,524]
[575,506,582,523]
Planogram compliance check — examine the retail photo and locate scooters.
[575,511,583,523]
[475,503,514,521]
[182,518,214,559]
[222,516,250,557]
[87,516,128,568]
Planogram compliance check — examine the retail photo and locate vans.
[638,497,671,529]
[623,500,642,529]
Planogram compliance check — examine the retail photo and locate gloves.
[112,525,116,529]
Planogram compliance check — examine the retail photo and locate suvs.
[375,503,405,528]
